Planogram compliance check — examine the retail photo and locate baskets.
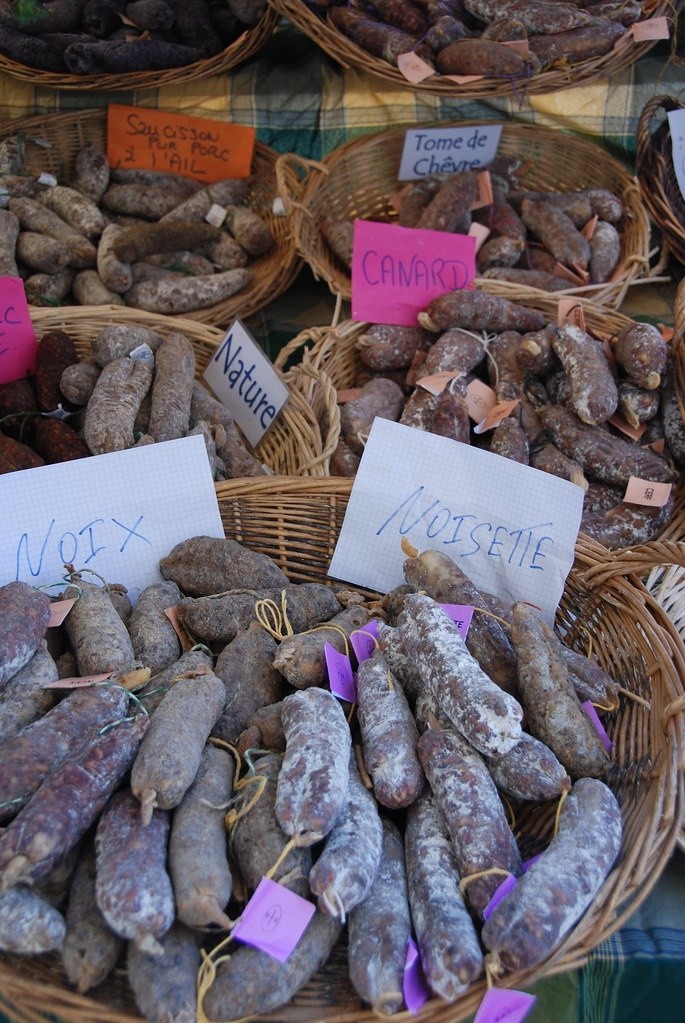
[274,288,685,562]
[0,475,685,1023]
[1,108,303,328]
[637,95,685,263]
[0,0,278,91]
[275,119,650,312]
[269,0,680,100]
[31,305,340,474]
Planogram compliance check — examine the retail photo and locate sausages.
[0,0,685,1023]
